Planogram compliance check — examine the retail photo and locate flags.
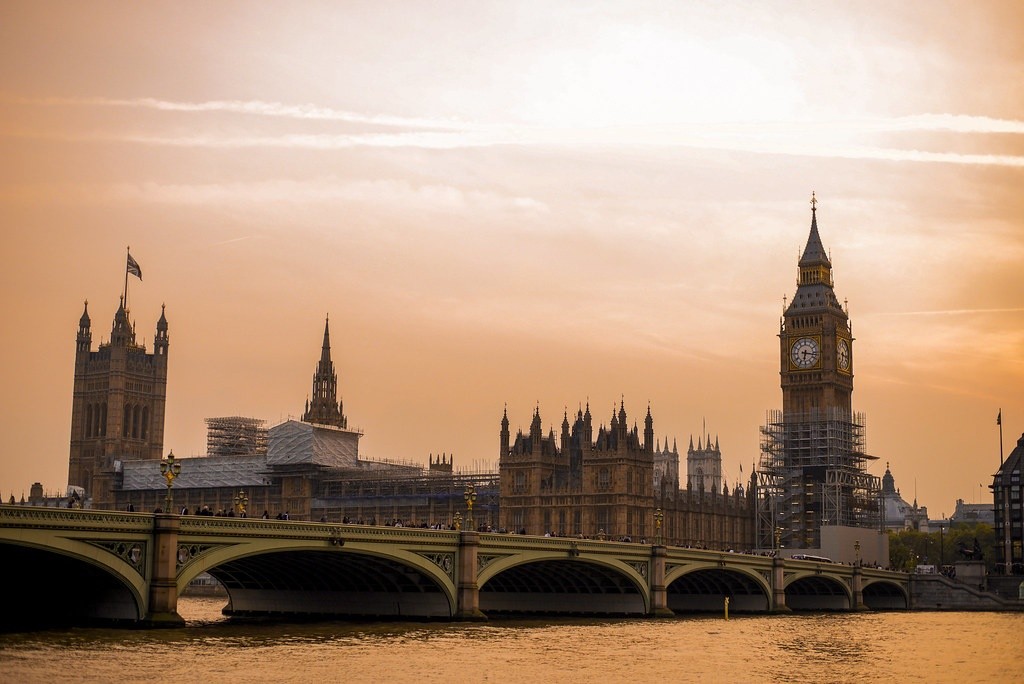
[996,413,1001,424]
[127,253,142,281]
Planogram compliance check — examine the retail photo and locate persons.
[477,523,508,533]
[675,542,708,550]
[520,528,526,535]
[941,566,957,580]
[67,496,75,508]
[126,501,134,512]
[275,511,290,520]
[343,514,350,524]
[180,505,189,515]
[544,531,567,537]
[790,553,910,574]
[576,531,584,539]
[240,510,246,518]
[194,505,235,517]
[641,538,645,543]
[721,545,776,558]
[392,519,456,530]
[357,517,364,525]
[262,510,269,519]
[617,534,631,542]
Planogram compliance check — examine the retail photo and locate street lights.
[909,549,915,568]
[696,540,701,549]
[233,488,248,516]
[774,526,784,557]
[939,527,945,571]
[160,448,181,514]
[925,536,933,564]
[854,539,861,560]
[653,508,663,534]
[464,484,478,530]
[596,529,606,540]
[453,511,463,530]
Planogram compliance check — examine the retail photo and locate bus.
[791,554,833,563]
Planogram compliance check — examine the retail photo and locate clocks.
[837,340,849,369]
[791,337,819,369]
